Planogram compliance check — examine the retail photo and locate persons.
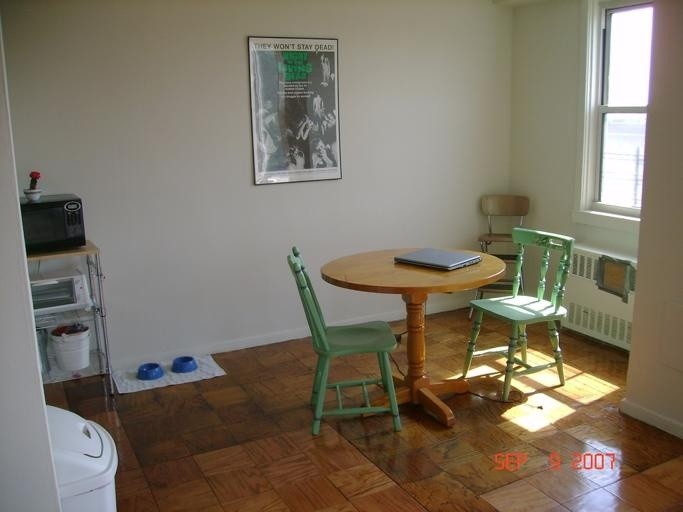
[256,52,339,174]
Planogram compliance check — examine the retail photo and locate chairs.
[462,193,576,402]
[286,247,403,437]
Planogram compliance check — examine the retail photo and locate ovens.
[29,266,92,316]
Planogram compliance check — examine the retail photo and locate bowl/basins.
[171,356,197,373]
[137,363,163,380]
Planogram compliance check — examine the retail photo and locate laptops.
[394,247,481,271]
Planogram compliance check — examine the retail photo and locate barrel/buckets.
[50,326,89,370]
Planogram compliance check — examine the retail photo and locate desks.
[319,247,507,430]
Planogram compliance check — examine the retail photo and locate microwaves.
[19,193,87,256]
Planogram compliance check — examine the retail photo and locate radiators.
[560,245,637,352]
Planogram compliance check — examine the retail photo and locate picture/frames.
[246,34,342,185]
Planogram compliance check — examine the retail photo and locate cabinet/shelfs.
[24,240,113,413]
